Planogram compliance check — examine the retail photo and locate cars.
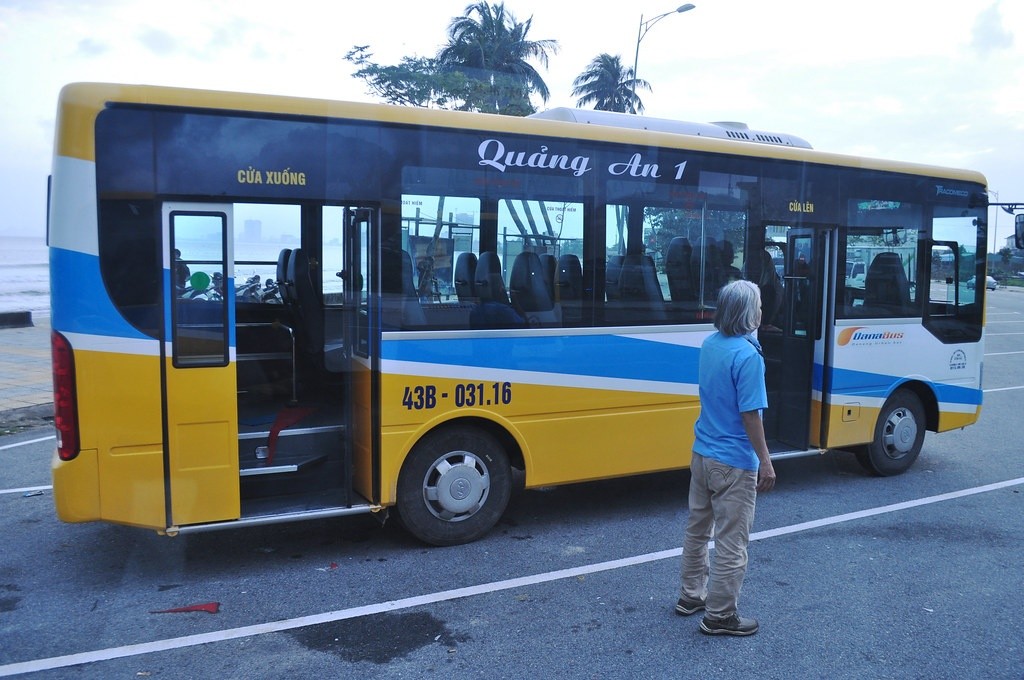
[966,275,997,292]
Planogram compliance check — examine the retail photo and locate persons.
[716,240,742,279]
[469,275,527,326]
[675,281,776,635]
[760,251,784,299]
[174,250,190,294]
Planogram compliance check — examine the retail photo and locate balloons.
[191,272,210,291]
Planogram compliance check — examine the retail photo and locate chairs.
[275,235,745,399]
[863,252,913,312]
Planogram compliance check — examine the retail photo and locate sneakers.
[675,597,706,615]
[699,612,758,635]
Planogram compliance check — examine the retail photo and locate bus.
[43,80,1024,547]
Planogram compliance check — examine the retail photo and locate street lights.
[629,1,696,114]
